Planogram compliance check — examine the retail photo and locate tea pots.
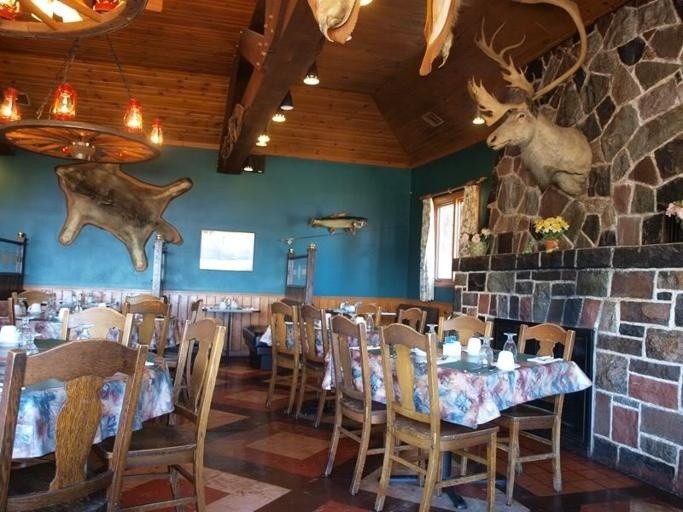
[339,301,362,312]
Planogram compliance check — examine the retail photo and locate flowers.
[532,216,570,240]
[665,200,683,222]
[460,228,490,258]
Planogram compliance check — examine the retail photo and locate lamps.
[0,0,149,41]
[243,62,320,173]
[0,34,165,164]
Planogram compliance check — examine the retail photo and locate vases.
[544,240,560,252]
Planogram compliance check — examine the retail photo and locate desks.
[202,305,260,362]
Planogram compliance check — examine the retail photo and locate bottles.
[14,298,28,316]
[220,297,240,310]
[14,318,36,353]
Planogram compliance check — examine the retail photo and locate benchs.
[244,325,269,370]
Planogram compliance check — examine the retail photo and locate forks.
[436,354,448,361]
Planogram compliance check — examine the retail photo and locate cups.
[495,351,514,371]
[28,304,40,312]
[41,288,124,320]
[371,333,379,347]
[468,338,480,352]
[445,336,455,344]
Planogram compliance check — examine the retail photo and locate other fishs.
[308,209,368,236]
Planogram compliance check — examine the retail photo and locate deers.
[467,0,593,197]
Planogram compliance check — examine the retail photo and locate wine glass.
[426,324,439,346]
[363,312,374,332]
[478,337,494,366]
[502,332,517,359]
[128,314,143,348]
[74,323,94,342]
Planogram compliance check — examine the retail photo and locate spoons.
[478,365,495,374]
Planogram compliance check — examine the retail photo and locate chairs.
[0,289,227,357]
[436,315,493,346]
[0,338,150,512]
[397,307,427,333]
[324,315,426,495]
[163,298,205,390]
[266,301,302,414]
[294,304,337,426]
[374,325,500,511]
[264,298,460,325]
[460,322,575,507]
[90,317,226,512]
